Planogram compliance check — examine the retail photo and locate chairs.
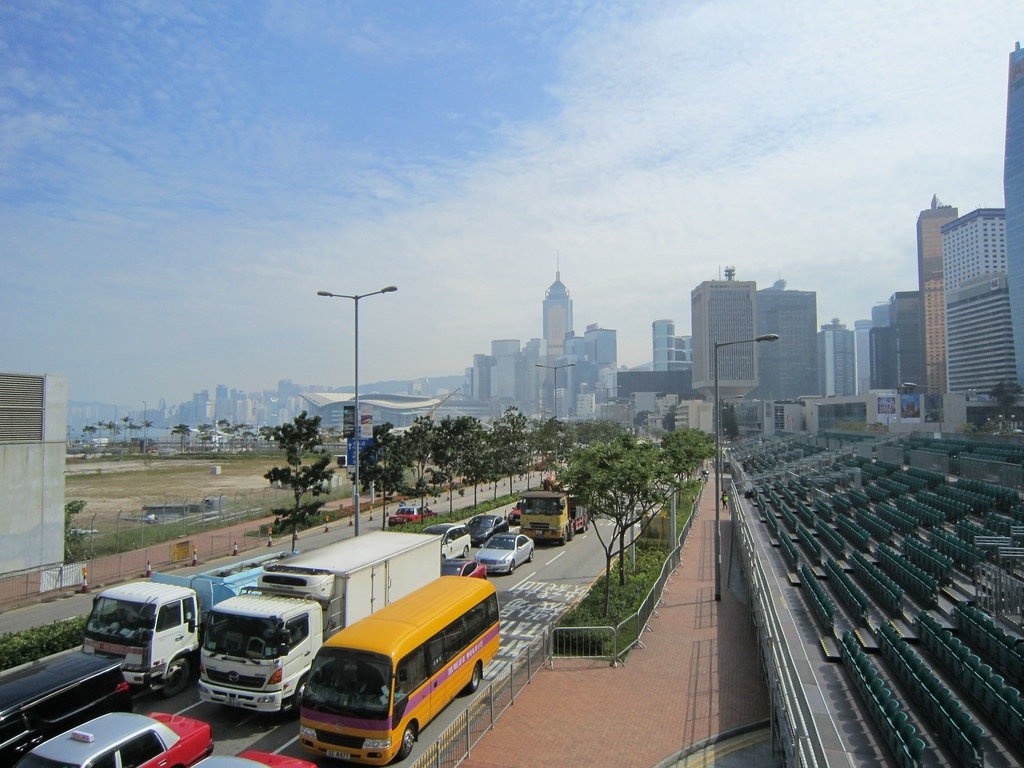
[723,430,1024,768]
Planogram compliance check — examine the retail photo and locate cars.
[388,503,438,527]
[474,533,534,575]
[12,712,214,768]
[189,750,316,768]
[465,515,509,547]
[508,501,521,525]
[442,558,487,579]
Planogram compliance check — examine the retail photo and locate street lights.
[720,395,742,491]
[714,332,779,599]
[595,386,621,420]
[903,382,942,433]
[318,286,397,535]
[536,363,576,476]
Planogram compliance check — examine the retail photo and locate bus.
[299,576,499,766]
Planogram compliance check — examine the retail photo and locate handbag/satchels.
[724,494,728,501]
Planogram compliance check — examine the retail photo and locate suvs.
[418,523,471,559]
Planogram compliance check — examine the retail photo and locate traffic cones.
[192,548,199,566]
[267,534,274,547]
[81,576,90,594]
[232,541,240,556]
[480,484,484,492]
[503,479,506,486]
[513,476,516,483]
[294,527,299,540]
[348,515,354,526]
[369,510,374,521]
[145,560,153,577]
[434,496,438,504]
[488,483,492,490]
[446,492,450,501]
[324,521,330,533]
[385,507,390,517]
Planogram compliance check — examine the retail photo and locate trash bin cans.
[702,470,709,483]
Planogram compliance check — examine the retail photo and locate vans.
[0,651,134,768]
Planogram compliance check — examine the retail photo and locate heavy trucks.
[199,530,442,720]
[518,489,589,545]
[81,548,302,697]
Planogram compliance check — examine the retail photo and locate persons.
[720,490,728,510]
